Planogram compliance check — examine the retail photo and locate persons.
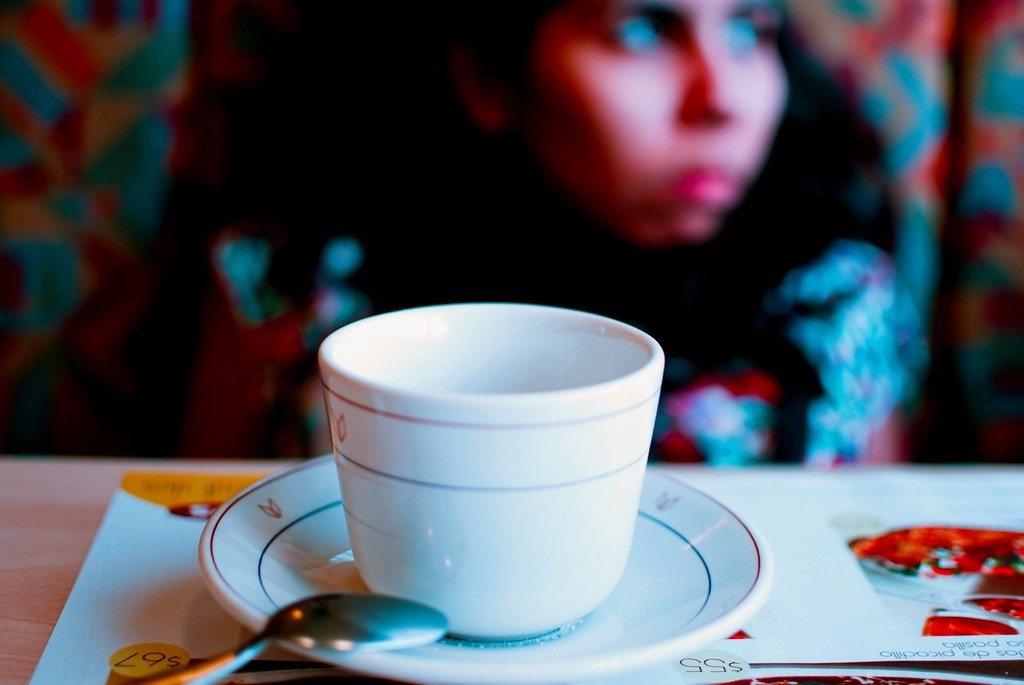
[185,2,932,467]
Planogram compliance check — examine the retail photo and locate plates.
[204,461,773,685]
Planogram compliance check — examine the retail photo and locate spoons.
[122,592,448,685]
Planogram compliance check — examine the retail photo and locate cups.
[319,302,664,639]
